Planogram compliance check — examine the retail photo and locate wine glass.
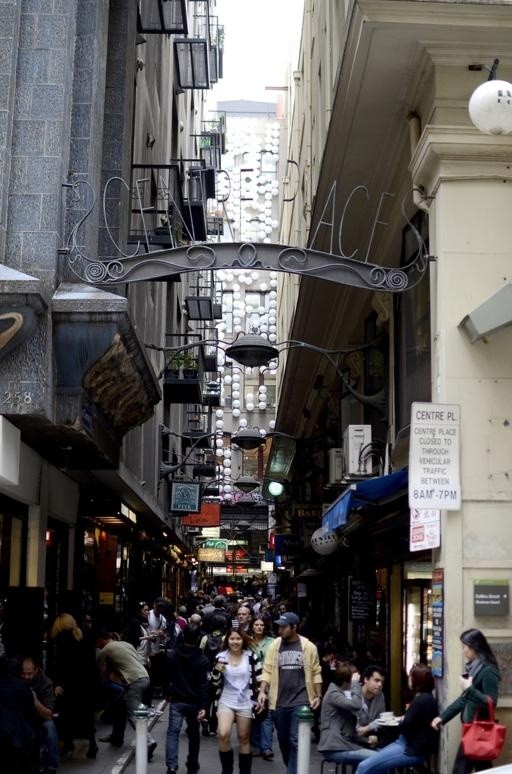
[218,655,229,672]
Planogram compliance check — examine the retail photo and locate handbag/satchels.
[250,700,268,725]
[460,695,506,761]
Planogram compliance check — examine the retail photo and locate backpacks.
[204,631,225,672]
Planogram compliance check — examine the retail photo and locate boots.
[218,747,233,774]
[239,752,252,774]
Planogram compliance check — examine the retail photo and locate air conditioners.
[318,444,340,489]
[334,425,371,479]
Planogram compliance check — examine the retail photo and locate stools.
[318,747,355,773]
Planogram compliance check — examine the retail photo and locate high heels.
[86,745,100,759]
[58,743,75,758]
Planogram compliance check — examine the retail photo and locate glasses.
[138,601,146,606]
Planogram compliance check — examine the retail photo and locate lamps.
[467,59,512,134]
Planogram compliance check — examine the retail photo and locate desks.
[373,703,407,732]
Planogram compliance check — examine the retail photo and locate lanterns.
[311,527,340,554]
[468,79,512,137]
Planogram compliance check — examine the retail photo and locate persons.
[2,576,439,772]
[430,627,499,774]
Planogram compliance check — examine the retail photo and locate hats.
[273,611,301,626]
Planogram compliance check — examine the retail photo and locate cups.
[379,711,393,720]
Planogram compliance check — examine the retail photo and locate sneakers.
[99,735,112,742]
[148,741,157,759]
[263,750,274,758]
[252,747,262,757]
[201,719,210,736]
[167,768,176,774]
